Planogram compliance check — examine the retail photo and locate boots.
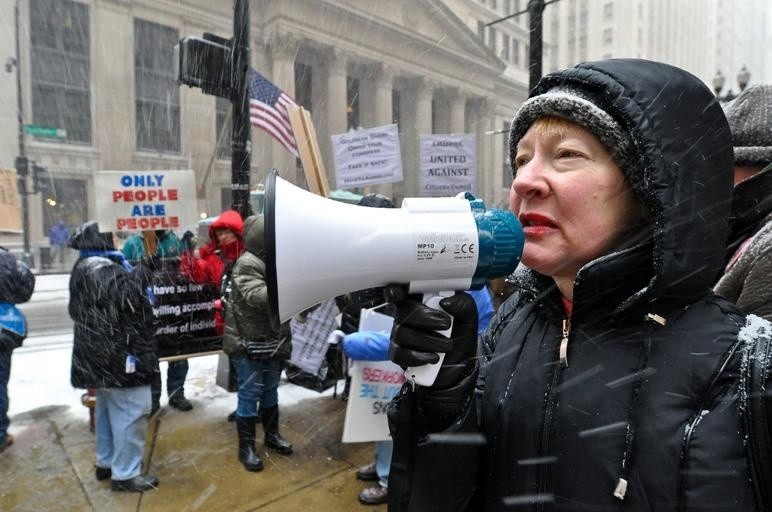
[236,415,264,471]
[265,406,292,457]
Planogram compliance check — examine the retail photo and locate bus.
[197,183,364,246]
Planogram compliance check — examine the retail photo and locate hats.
[726,82,771,166]
[509,86,641,204]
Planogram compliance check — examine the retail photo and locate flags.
[246,65,302,159]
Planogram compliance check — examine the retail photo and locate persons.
[67,219,161,491]
[335,192,516,505]
[180,209,246,332]
[386,59,772,512]
[3,247,34,450]
[119,229,191,418]
[48,219,68,260]
[711,83,772,320]
[222,215,295,471]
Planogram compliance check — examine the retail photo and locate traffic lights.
[16,157,29,190]
[32,164,48,194]
[180,35,238,93]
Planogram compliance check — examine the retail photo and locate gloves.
[386,292,479,431]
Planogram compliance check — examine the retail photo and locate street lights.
[709,63,751,104]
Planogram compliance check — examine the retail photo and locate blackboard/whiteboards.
[127,271,226,363]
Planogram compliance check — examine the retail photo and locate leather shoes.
[356,461,379,479]
[95,466,158,493]
[169,396,192,410]
[359,483,385,504]
[148,402,159,417]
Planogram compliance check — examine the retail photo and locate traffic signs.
[24,124,66,139]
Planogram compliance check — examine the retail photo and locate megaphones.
[263,169,527,387]
